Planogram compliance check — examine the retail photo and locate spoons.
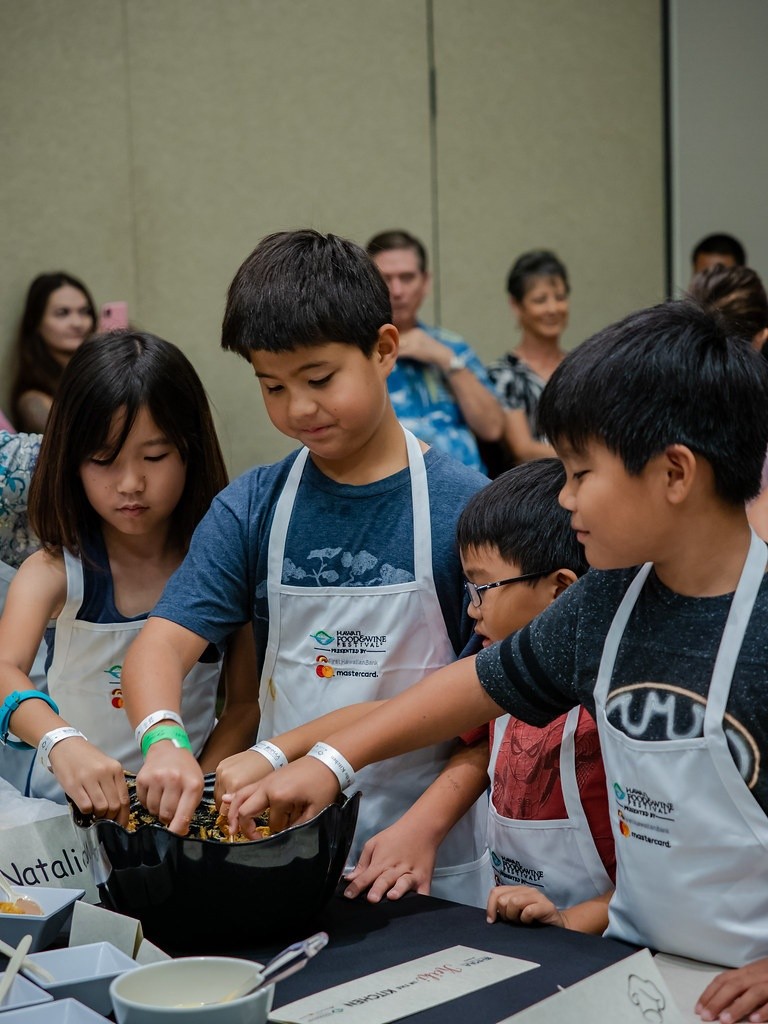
[0,871,31,906]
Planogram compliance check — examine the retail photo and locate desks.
[61,874,752,1024]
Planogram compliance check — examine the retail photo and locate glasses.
[463,571,555,608]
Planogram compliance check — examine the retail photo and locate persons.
[218,289,766,1024]
[0,327,264,832]
[8,270,101,434]
[684,233,768,543]
[120,228,498,913]
[212,457,617,935]
[473,242,578,477]
[365,232,510,480]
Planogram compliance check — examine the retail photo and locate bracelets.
[250,740,289,770]
[135,709,186,748]
[36,727,89,773]
[309,743,355,792]
[142,726,194,759]
[442,356,466,377]
[0,689,60,752]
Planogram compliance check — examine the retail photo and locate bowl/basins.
[109,955,276,1024]
[70,765,363,963]
[0,886,87,962]
[0,941,144,1024]
